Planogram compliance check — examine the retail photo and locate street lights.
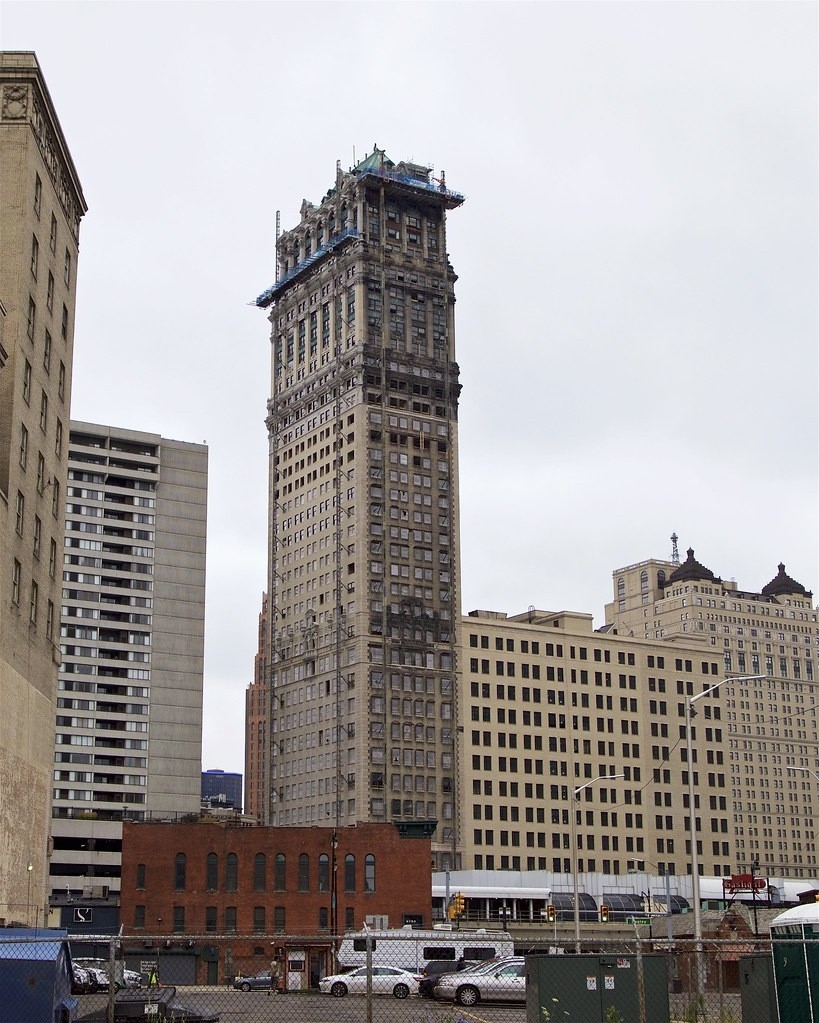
[750,857,761,939]
[631,857,677,996]
[639,892,653,953]
[330,829,339,973]
[570,774,626,954]
[685,674,763,1015]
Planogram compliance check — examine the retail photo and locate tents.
[768,903,819,1023]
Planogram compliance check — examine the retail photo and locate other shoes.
[273,992,277,996]
[268,989,271,995]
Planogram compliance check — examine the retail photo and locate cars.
[435,956,526,1006]
[233,969,273,992]
[420,961,480,999]
[72,961,141,995]
[318,964,419,999]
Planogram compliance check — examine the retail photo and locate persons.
[422,970,431,978]
[147,966,159,990]
[588,948,594,954]
[600,947,606,953]
[456,957,466,972]
[267,956,279,996]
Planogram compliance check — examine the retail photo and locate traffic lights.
[450,891,465,919]
[548,907,554,922]
[815,893,819,904]
[600,905,609,922]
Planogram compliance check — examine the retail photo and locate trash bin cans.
[104,986,220,1023]
[0,927,79,1023]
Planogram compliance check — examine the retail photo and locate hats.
[495,953,501,958]
